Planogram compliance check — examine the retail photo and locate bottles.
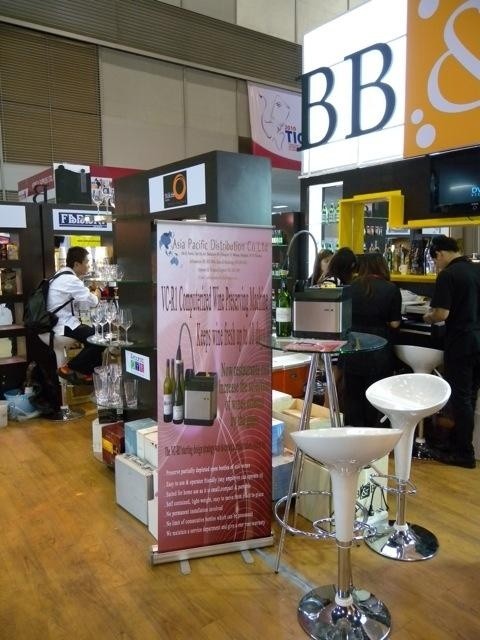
[383,238,437,276]
[320,199,339,251]
[162,357,185,424]
[275,269,292,338]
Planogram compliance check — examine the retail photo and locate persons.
[341,248,403,428]
[306,249,333,286]
[37,245,104,387]
[421,233,480,468]
[317,246,357,408]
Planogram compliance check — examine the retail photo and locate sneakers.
[57,367,93,385]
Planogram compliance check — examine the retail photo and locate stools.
[38,333,86,423]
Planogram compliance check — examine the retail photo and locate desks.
[256,330,388,575]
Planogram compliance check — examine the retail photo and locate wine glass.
[90,256,139,412]
[89,186,115,216]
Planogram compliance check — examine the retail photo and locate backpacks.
[23,271,74,358]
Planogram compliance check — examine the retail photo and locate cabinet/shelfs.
[0,201,44,402]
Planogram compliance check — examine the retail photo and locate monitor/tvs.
[121,345,156,391]
[428,143,480,215]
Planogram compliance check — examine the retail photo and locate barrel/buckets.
[4,384,35,422]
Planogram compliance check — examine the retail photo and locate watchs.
[97,287,103,292]
